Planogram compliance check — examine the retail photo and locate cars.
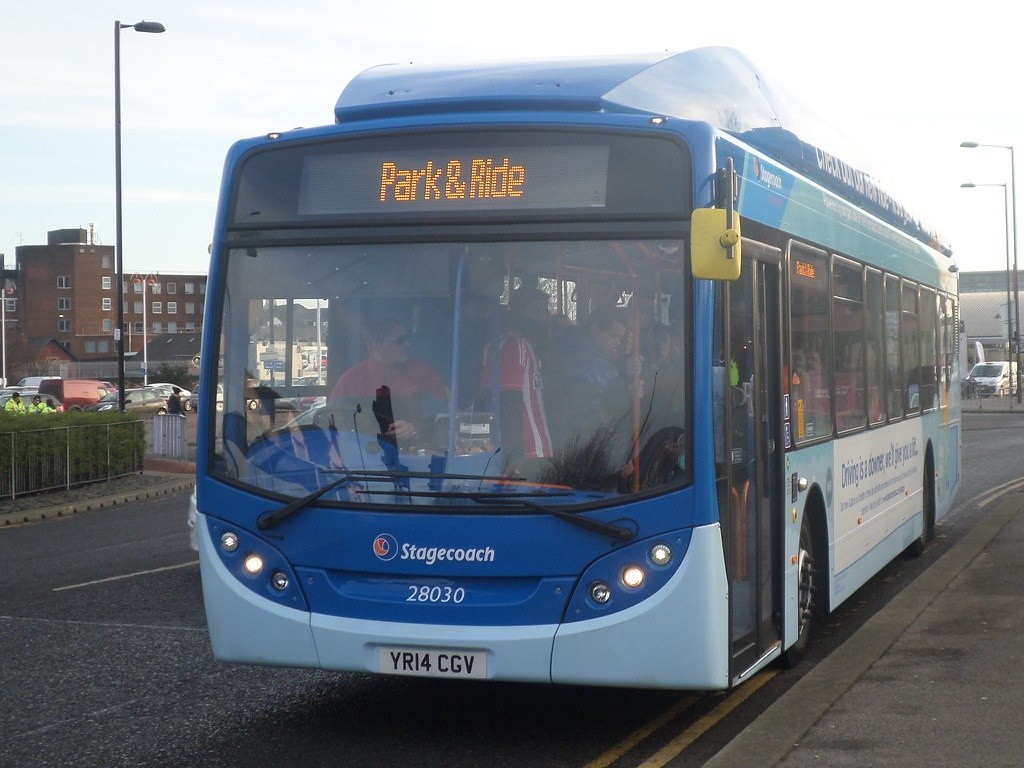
[257,379,300,411]
[294,376,326,409]
[190,384,224,413]
[312,355,328,371]
[0,386,39,395]
[136,383,192,411]
[83,389,168,419]
[38,379,111,414]
[17,376,62,386]
[245,379,259,410]
[101,381,116,393]
[0,393,64,413]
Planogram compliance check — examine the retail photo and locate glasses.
[382,334,410,344]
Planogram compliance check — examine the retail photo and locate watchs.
[412,431,418,441]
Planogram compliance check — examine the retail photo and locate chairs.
[329,299,960,482]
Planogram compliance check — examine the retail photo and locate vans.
[966,361,1017,398]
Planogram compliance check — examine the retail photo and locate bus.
[187,45,965,694]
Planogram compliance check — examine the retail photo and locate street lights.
[130,271,158,385]
[961,183,1013,403]
[113,19,166,414]
[960,140,1022,404]
[2,288,13,388]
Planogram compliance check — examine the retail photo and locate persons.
[326,318,450,457]
[168,386,186,416]
[482,284,684,476]
[4,392,26,415]
[42,398,57,414]
[28,395,42,414]
[805,349,824,371]
